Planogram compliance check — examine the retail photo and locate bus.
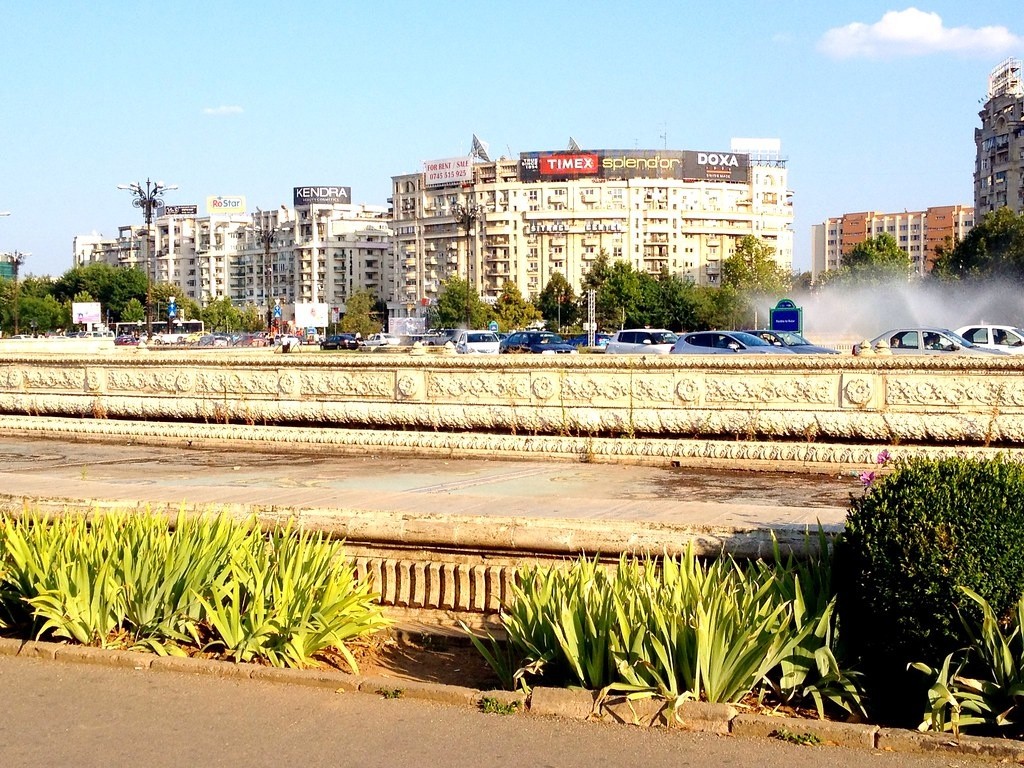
[422,328,465,347]
[116,321,204,346]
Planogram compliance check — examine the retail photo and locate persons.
[925,334,944,350]
[891,337,899,347]
[540,338,549,343]
[999,332,1009,345]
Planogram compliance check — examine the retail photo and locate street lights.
[117,177,179,345]
[446,194,493,326]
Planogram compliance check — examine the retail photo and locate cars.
[11,330,115,340]
[199,326,328,348]
[455,330,502,355]
[113,335,140,346]
[567,329,696,355]
[320,335,359,351]
[669,331,797,354]
[744,328,843,354]
[852,325,1011,356]
[499,330,580,355]
[360,333,400,347]
[186,330,212,345]
[939,324,1024,356]
